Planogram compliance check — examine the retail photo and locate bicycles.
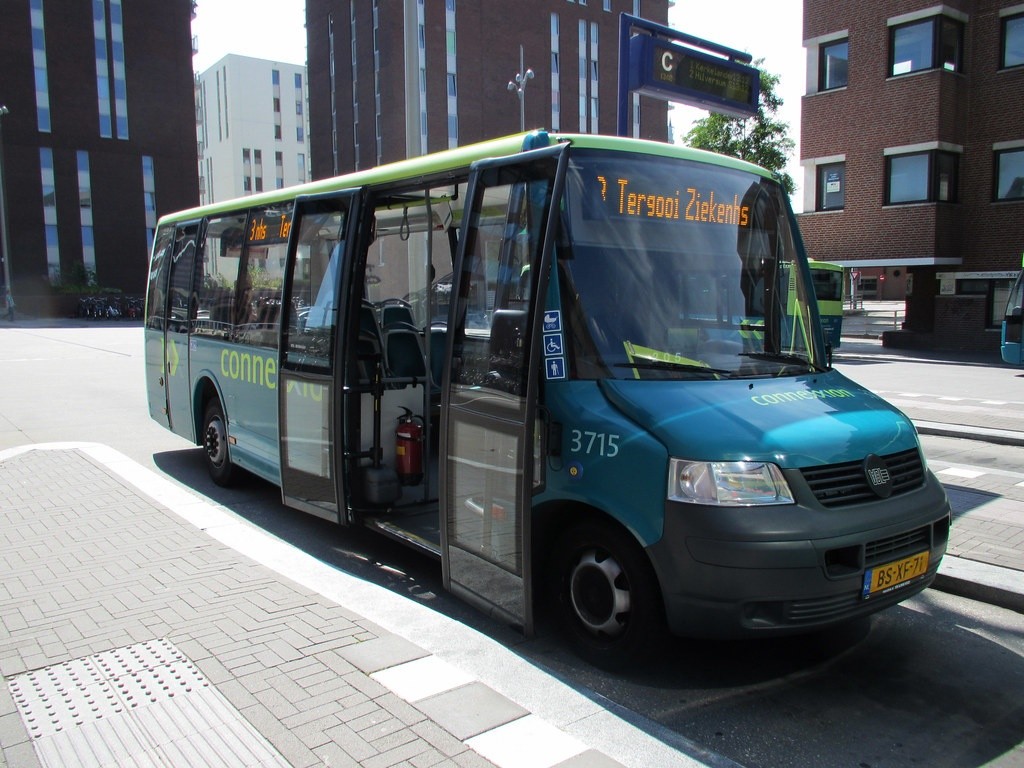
[78,295,146,321]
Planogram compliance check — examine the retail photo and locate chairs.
[208,294,251,331]
[298,298,470,400]
[255,297,296,334]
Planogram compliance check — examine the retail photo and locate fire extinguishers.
[392,404,427,487]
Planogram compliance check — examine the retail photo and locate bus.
[676,262,844,371]
[142,131,955,678]
[1000,252,1024,366]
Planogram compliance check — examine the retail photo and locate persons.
[3,290,15,321]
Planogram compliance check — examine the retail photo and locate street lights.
[507,44,534,134]
